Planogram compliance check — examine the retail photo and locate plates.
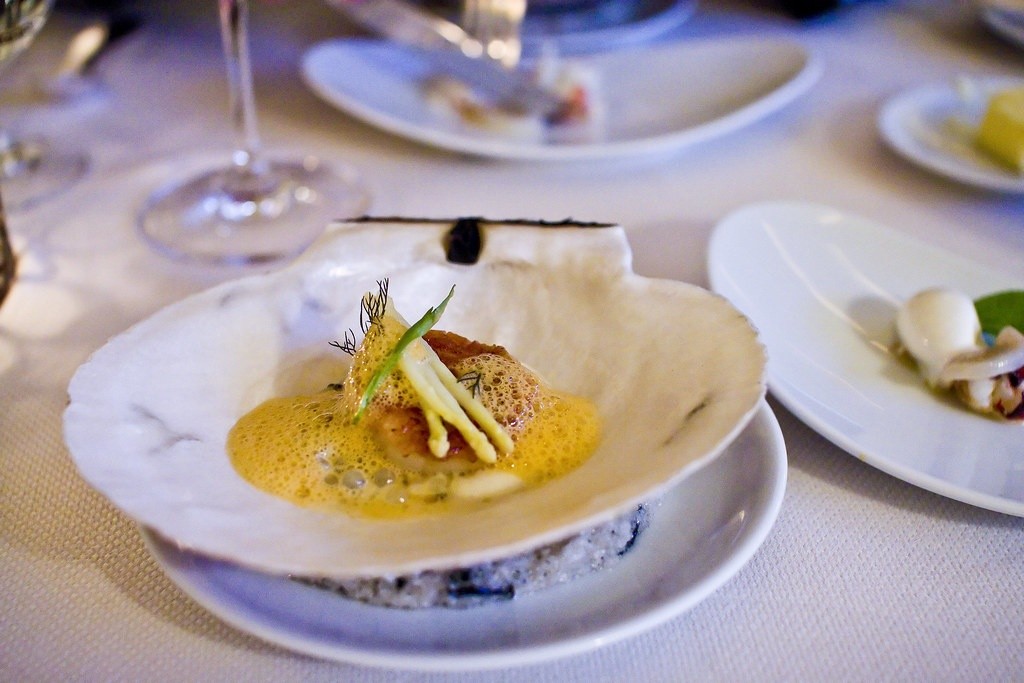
[136,397,789,672]
[706,199,1024,519]
[298,35,824,161]
[876,72,1024,194]
[327,0,701,51]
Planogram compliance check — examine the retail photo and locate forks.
[461,0,529,70]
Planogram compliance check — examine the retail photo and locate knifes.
[330,0,559,104]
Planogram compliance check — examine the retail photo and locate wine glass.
[0,0,92,213]
[138,0,376,266]
